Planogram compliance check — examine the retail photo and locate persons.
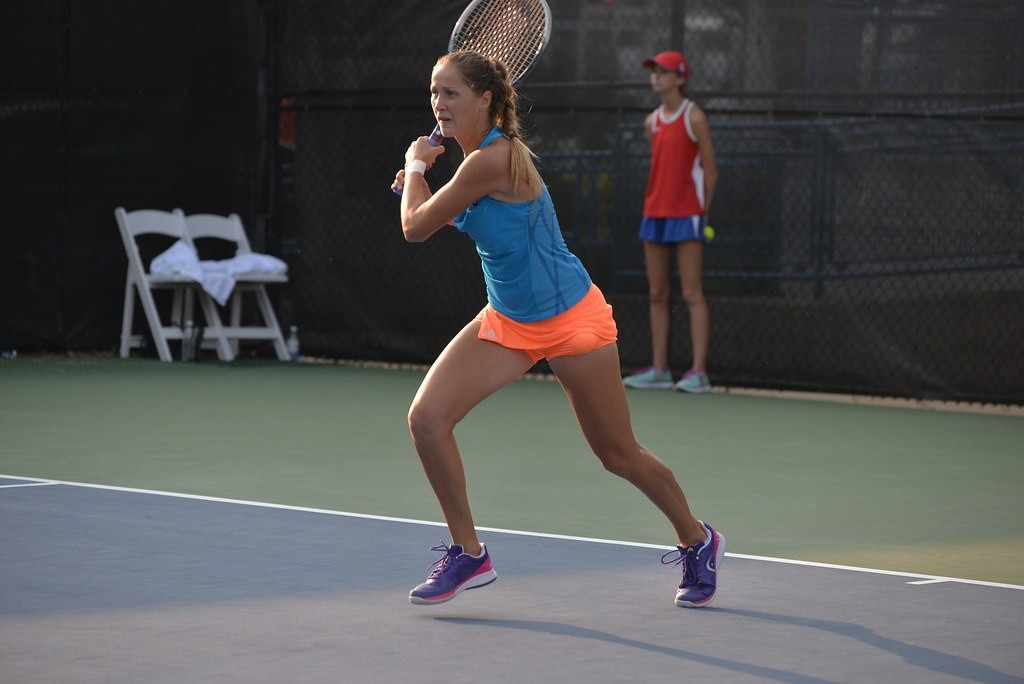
[391,50,726,607]
[622,52,717,393]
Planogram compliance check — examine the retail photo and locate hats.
[642,50,689,78]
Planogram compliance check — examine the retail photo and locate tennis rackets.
[393,1,553,196]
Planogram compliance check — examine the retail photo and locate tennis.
[704,226,714,240]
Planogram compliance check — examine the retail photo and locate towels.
[148,240,291,306]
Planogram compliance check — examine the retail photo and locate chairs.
[115,206,235,364]
[169,213,292,362]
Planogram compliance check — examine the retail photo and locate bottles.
[286,327,299,362]
[181,321,194,361]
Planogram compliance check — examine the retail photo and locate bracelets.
[405,161,427,176]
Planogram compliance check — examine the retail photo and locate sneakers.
[675,369,713,394]
[623,366,675,391]
[660,520,726,608]
[408,539,498,606]
